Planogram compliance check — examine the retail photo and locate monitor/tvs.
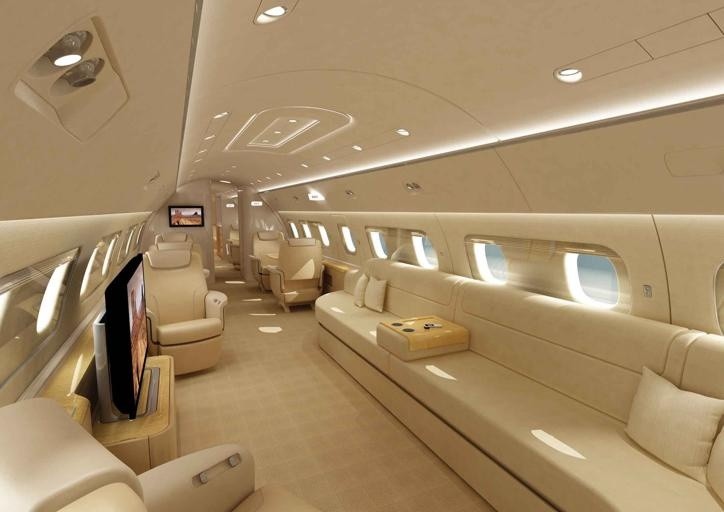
[168,205,205,227]
[105,254,150,417]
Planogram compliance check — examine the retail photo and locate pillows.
[362,276,389,314]
[621,364,723,486]
[704,421,723,504]
[352,273,368,311]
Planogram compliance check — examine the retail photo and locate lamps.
[59,54,105,91]
[41,28,95,70]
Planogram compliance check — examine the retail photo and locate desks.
[376,314,473,365]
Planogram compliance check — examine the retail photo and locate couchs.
[267,235,326,315]
[229,239,240,271]
[147,230,210,296]
[228,224,239,250]
[246,229,289,294]
[0,395,327,512]
[312,255,723,512]
[136,246,230,381]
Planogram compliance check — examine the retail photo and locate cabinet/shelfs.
[85,351,180,480]
